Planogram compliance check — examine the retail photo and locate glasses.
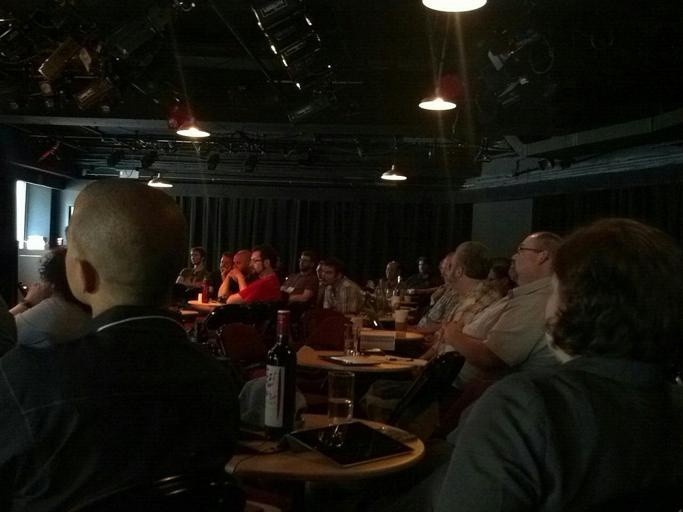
[299,258,311,262]
[252,258,261,261]
[518,246,542,254]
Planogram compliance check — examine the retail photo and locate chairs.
[386,351,465,428]
[64,472,239,511]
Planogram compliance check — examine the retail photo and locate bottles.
[264,310,297,435]
[202,274,209,304]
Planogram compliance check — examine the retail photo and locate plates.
[331,355,382,365]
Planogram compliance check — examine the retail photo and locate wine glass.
[319,371,354,446]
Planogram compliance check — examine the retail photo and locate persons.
[439,230,562,442]
[174,245,215,290]
[8,248,91,348]
[0,176,241,512]
[390,216,680,512]
[210,248,281,312]
[285,246,512,396]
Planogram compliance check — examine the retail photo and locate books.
[288,421,414,469]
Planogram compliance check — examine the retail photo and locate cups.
[395,309,408,332]
[343,324,360,357]
[198,293,202,303]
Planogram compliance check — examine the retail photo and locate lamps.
[422,0,487,13]
[417,10,456,111]
[380,135,408,181]
[176,113,210,139]
[147,173,173,188]
[250,1,336,93]
